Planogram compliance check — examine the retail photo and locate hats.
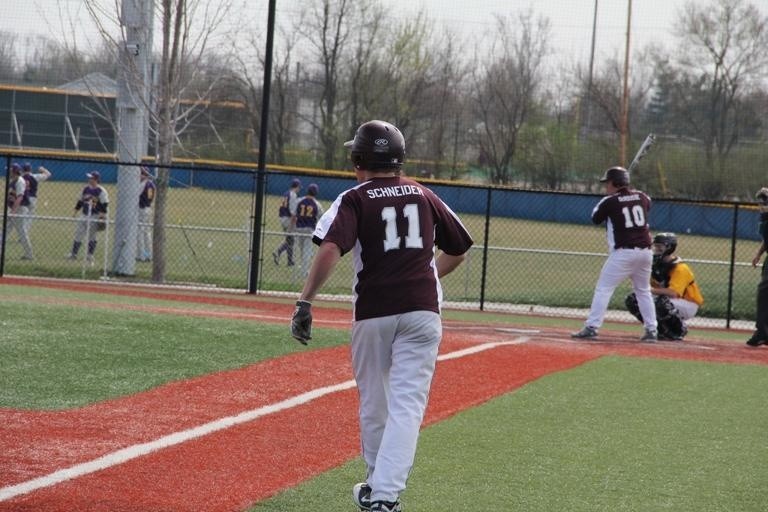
[293,179,300,184]
[13,163,31,171]
[86,171,99,179]
[309,184,318,193]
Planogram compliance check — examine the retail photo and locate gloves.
[291,300,312,345]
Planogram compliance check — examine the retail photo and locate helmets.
[344,119,406,166]
[601,167,628,185]
[654,232,677,256]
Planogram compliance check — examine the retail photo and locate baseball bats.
[626,133,655,175]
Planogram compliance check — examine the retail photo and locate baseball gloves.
[650,259,667,282]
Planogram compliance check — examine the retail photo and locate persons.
[747,188,768,349]
[63,171,110,261]
[17,162,51,242]
[625,234,704,341]
[570,167,659,341]
[291,119,474,512]
[289,184,325,277]
[0,163,33,259]
[136,167,155,262]
[272,177,297,266]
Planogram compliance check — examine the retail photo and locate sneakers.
[272,251,280,264]
[353,483,401,512]
[747,334,768,345]
[641,328,658,341]
[572,327,598,337]
